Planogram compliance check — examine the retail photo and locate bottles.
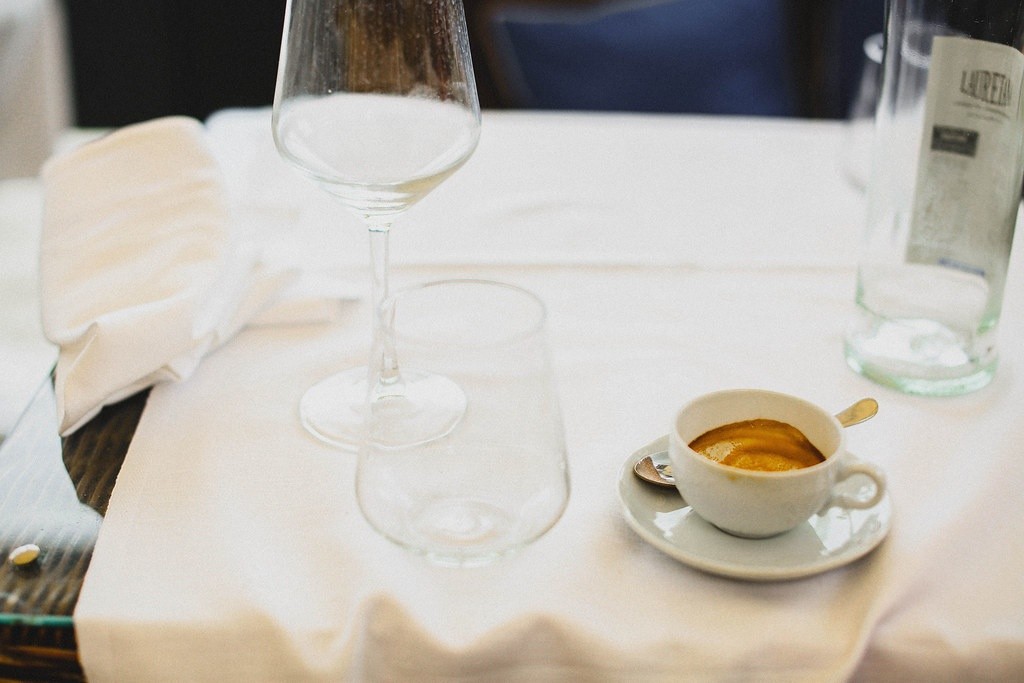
[838,0,1023,396]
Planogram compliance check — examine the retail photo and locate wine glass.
[266,0,483,452]
[353,276,570,571]
[842,31,887,262]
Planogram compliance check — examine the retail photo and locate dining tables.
[2,110,1024,683]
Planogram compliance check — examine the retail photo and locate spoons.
[634,396,880,489]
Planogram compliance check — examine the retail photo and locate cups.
[667,385,889,541]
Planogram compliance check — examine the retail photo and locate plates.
[615,431,898,582]
